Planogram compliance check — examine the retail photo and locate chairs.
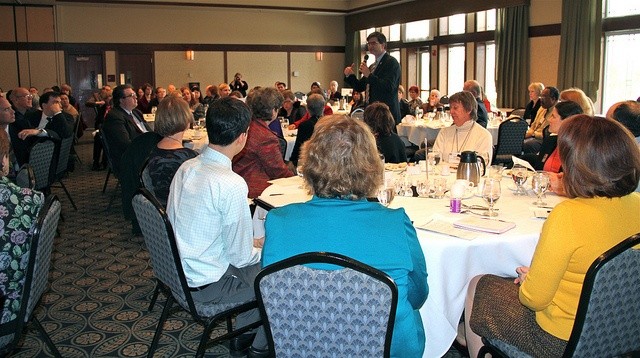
[130,187,265,357]
[74,112,82,133]
[15,162,36,189]
[25,137,65,238]
[475,232,640,357]
[95,106,99,117]
[98,123,122,211]
[17,190,77,358]
[494,116,529,165]
[55,135,77,212]
[77,101,81,110]
[253,252,397,357]
[350,108,365,119]
[125,132,161,188]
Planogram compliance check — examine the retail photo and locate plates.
[385,163,408,172]
[502,169,513,179]
[473,187,485,198]
[452,192,473,200]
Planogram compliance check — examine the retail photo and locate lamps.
[315,51,324,62]
[185,50,194,59]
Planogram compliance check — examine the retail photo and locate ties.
[366,61,378,104]
[45,117,52,128]
[131,113,144,134]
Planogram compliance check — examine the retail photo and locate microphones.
[358,55,369,75]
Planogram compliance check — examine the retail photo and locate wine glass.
[377,178,396,207]
[492,110,498,120]
[281,118,289,138]
[438,113,448,124]
[428,148,442,175]
[532,171,549,207]
[415,109,424,120]
[478,152,490,178]
[500,111,507,122]
[487,112,494,126]
[198,118,206,134]
[512,163,528,196]
[192,120,201,137]
[490,165,504,182]
[278,116,285,128]
[483,178,502,217]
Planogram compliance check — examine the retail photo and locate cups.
[450,180,475,196]
[387,176,447,197]
[477,180,486,192]
[297,159,307,177]
[440,163,451,176]
[418,161,436,171]
[449,198,461,213]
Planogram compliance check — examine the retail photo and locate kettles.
[456,151,486,186]
[435,106,443,120]
[444,106,452,120]
[338,99,346,110]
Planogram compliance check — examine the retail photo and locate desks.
[327,100,353,115]
[143,112,206,124]
[180,127,297,162]
[397,113,505,148]
[509,106,525,116]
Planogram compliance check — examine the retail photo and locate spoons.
[460,210,489,217]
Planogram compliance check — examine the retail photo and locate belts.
[189,283,213,292]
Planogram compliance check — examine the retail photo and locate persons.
[203,84,220,104]
[29,87,41,97]
[60,93,80,125]
[104,84,156,234]
[542,102,584,174]
[0,128,44,347]
[191,85,207,105]
[429,90,493,166]
[60,83,80,113]
[278,90,306,125]
[408,85,423,109]
[92,91,113,171]
[24,92,74,138]
[414,80,488,162]
[454,114,639,357]
[418,89,445,120]
[180,86,205,122]
[306,82,320,96]
[0,96,16,158]
[606,102,640,193]
[289,94,326,167]
[85,86,111,114]
[260,113,428,358]
[230,86,294,198]
[24,103,24,106]
[343,32,401,133]
[326,80,343,102]
[398,85,410,118]
[167,84,181,97]
[229,73,248,98]
[148,87,168,114]
[520,88,592,170]
[139,96,201,208]
[0,89,60,169]
[287,88,333,130]
[9,87,43,128]
[523,82,545,127]
[218,82,231,99]
[137,84,157,114]
[523,86,559,154]
[349,89,368,114]
[136,87,145,101]
[164,99,270,356]
[480,86,490,112]
[364,102,408,162]
[277,83,286,93]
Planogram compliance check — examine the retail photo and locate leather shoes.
[247,345,269,358]
[229,331,256,357]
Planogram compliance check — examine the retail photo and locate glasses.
[14,94,33,99]
[121,92,136,98]
[367,41,381,45]
